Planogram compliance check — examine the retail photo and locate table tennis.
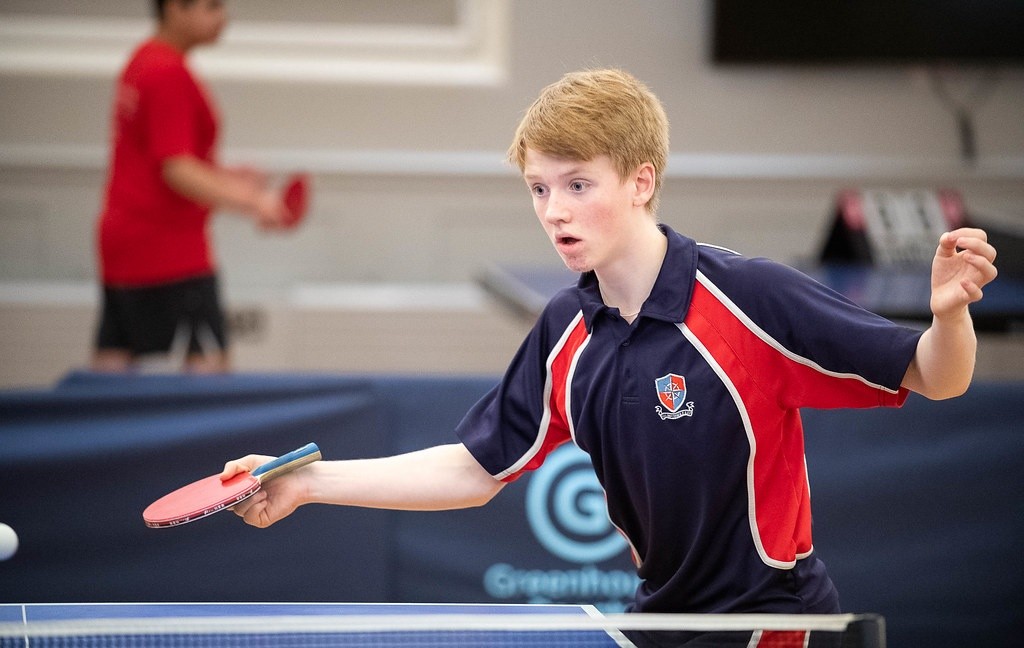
[0,522,18,562]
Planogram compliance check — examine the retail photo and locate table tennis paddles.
[283,174,308,223]
[142,441,325,530]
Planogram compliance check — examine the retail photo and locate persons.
[221,68,998,648]
[83,0,295,377]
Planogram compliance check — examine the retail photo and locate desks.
[0,597,646,648]
[474,258,1023,353]
